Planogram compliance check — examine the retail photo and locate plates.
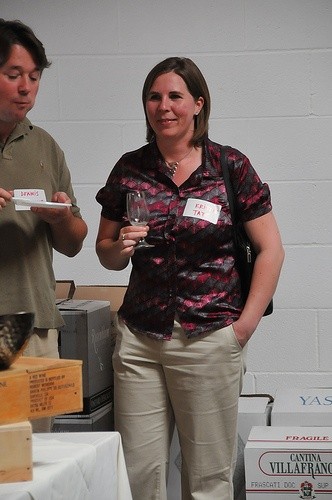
[10,197,72,208]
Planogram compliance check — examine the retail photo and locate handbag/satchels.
[222,147,276,318]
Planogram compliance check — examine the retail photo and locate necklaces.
[157,145,194,177]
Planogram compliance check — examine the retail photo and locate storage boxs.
[53,402,113,432]
[54,279,76,299]
[71,284,127,352]
[271,389,332,425]
[0,356,83,425]
[243,425,331,500]
[167,397,271,500]
[55,299,113,398]
[0,421,33,483]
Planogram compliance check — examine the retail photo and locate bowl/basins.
[0,311,35,370]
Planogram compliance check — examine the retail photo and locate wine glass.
[127,192,155,249]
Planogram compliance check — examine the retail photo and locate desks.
[1,431,133,500]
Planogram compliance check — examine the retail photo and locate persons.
[95,56,285,500]
[0,17,87,432]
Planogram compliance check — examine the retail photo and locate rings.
[121,233,126,240]
